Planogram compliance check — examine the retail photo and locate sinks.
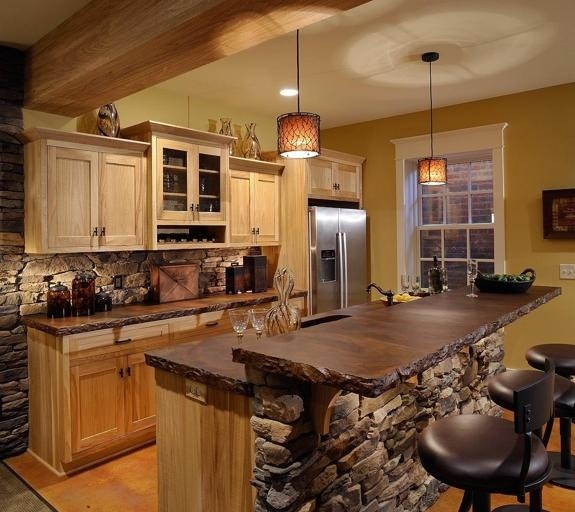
[297,313,352,329]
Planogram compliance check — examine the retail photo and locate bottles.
[43,271,112,319]
[241,122,263,160]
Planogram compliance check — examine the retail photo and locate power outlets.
[183,377,208,404]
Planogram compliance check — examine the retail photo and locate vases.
[241,122,266,162]
[232,122,243,157]
[97,103,121,140]
[208,118,218,134]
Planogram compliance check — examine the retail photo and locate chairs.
[418,358,559,511]
[525,341,575,490]
[487,368,574,511]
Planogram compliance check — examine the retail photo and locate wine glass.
[464,258,481,299]
[248,309,269,341]
[228,309,248,346]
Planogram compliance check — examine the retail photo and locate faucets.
[365,282,395,307]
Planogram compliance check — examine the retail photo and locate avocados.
[482,273,530,282]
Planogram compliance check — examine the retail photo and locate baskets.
[475,268,535,293]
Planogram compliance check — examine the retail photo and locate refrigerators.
[308,204,369,314]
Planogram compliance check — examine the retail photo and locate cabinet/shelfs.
[254,296,306,333]
[281,152,366,202]
[122,121,231,248]
[171,305,254,348]
[25,320,170,478]
[25,123,148,254]
[223,154,286,247]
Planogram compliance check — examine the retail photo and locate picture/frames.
[540,188,574,240]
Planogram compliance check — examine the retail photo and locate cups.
[411,274,420,296]
[402,273,409,292]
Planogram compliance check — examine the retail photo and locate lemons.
[393,293,412,302]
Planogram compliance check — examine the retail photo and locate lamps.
[274,26,324,158]
[415,53,450,185]
